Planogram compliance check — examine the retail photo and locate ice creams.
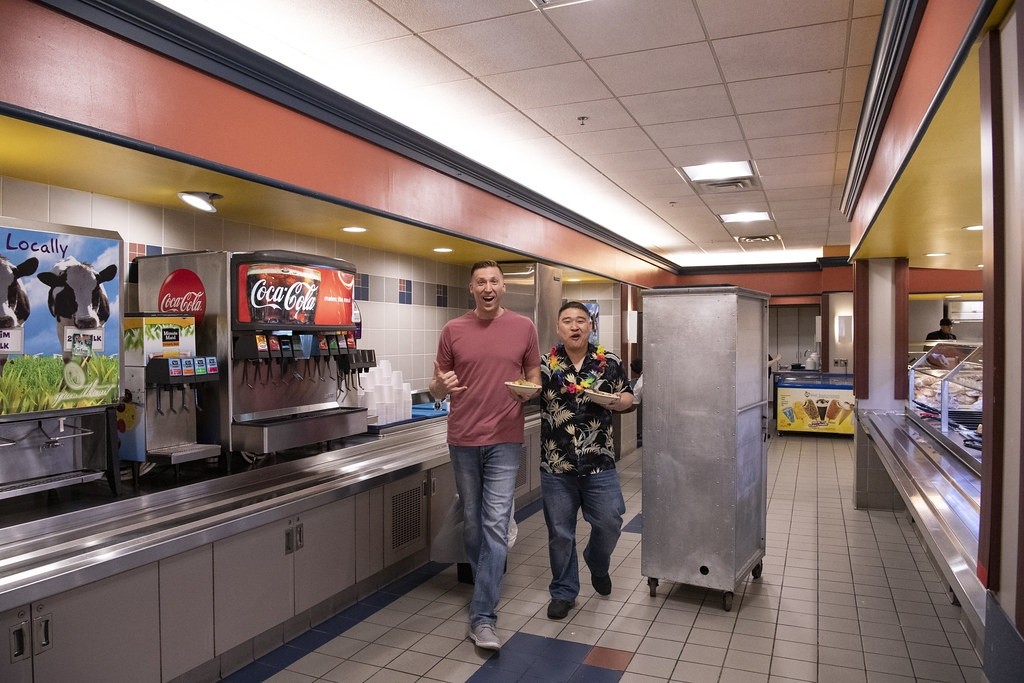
[838,402,852,425]
[816,399,829,421]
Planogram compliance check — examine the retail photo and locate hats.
[940,318,953,326]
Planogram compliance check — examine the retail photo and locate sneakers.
[547,596,575,619]
[469,618,502,650]
[583,548,612,595]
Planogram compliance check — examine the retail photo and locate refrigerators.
[778,373,855,436]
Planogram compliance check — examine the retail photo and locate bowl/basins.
[584,388,619,404]
[505,381,542,396]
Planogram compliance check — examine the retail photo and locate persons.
[539,300,635,620]
[429,259,543,651]
[613,357,644,415]
[923,318,956,352]
[768,353,782,379]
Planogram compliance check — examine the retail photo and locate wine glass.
[817,402,827,425]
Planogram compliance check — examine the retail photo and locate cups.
[247,265,322,324]
[357,359,412,426]
